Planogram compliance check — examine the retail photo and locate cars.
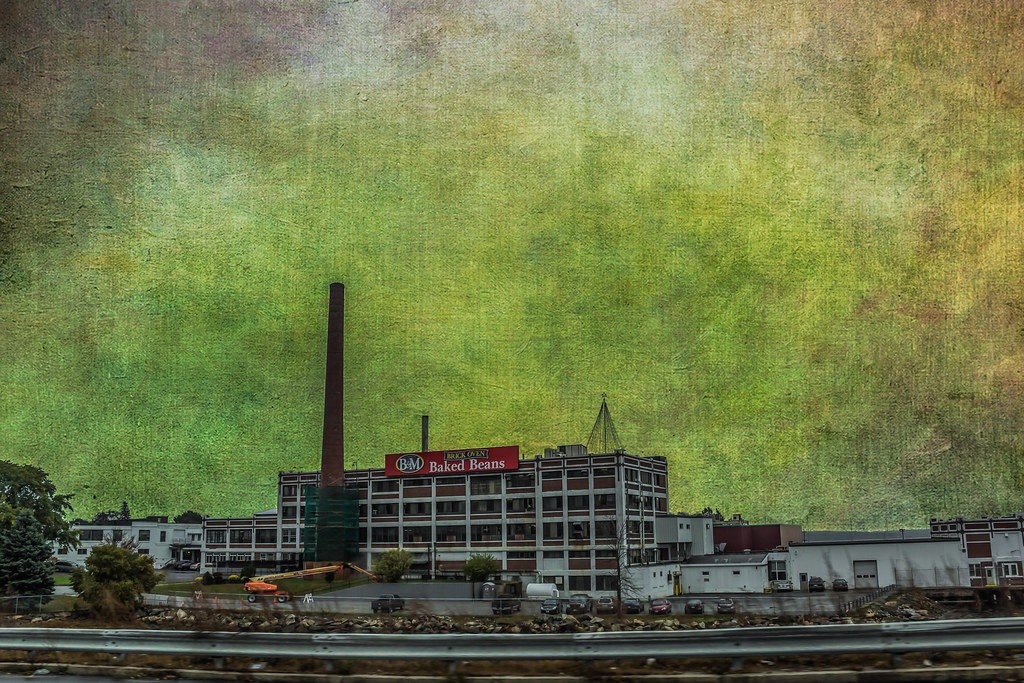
[649,598,672,615]
[777,580,793,592]
[622,598,644,614]
[832,579,848,592]
[541,599,563,614]
[190,562,200,570]
[685,599,704,614]
[54,560,78,573]
[174,560,195,570]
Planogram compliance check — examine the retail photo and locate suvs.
[597,596,617,614]
[809,577,825,592]
[717,599,734,613]
[566,594,594,613]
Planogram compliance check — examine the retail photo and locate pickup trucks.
[492,594,521,614]
[371,594,405,613]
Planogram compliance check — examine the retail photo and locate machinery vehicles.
[243,561,377,603]
